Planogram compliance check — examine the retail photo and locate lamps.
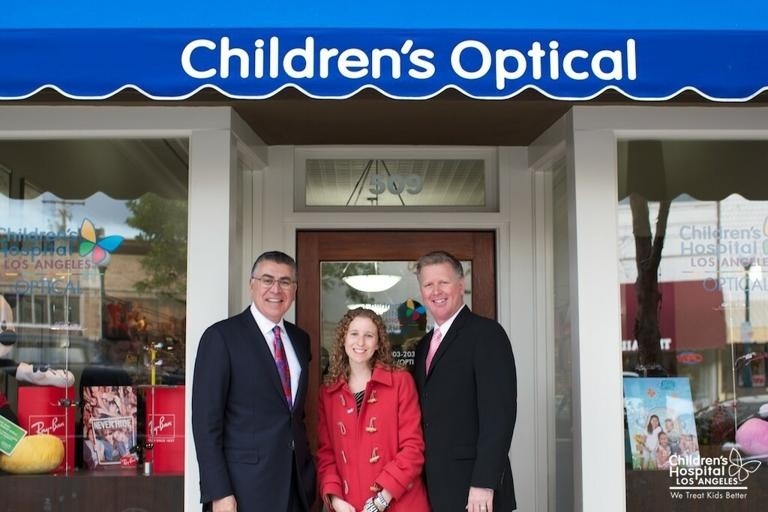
[336,157,424,292]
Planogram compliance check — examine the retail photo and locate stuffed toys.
[722,402,768,463]
[0,290,76,473]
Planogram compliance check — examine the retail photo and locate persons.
[656,431,672,470]
[663,418,680,456]
[406,250,518,512]
[191,250,318,512]
[316,307,431,512]
[642,415,662,471]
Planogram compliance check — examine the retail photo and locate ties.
[271,325,293,412]
[425,329,441,378]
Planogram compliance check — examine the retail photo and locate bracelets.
[363,492,389,512]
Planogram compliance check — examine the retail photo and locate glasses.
[251,276,298,290]
